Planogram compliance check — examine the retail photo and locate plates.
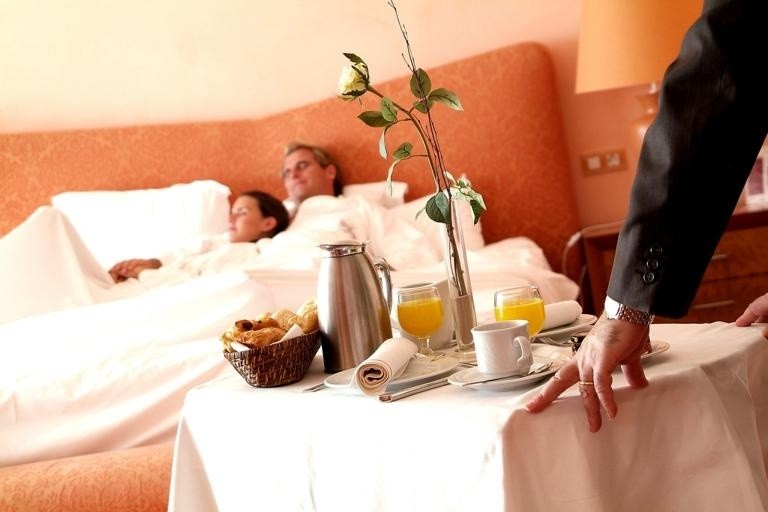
[446,357,561,394]
[640,338,671,361]
[533,313,600,338]
[323,356,460,392]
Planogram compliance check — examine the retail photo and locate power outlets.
[599,146,630,174]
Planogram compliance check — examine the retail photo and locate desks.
[164,296,768,512]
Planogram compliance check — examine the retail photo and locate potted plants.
[337,2,483,354]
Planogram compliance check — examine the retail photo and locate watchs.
[603,293,655,327]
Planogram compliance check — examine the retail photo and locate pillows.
[269,180,407,216]
[49,176,226,278]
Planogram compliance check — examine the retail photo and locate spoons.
[461,361,554,387]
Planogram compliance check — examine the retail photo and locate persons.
[107,140,346,285]
[522,0,768,434]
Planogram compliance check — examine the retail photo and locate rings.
[578,381,594,386]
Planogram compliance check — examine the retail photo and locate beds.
[0,43,581,512]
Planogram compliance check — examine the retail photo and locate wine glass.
[396,286,447,361]
[493,283,547,338]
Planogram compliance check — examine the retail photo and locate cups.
[392,278,454,349]
[470,319,534,380]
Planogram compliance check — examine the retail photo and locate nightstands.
[578,208,768,324]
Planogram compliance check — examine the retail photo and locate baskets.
[224,330,322,387]
